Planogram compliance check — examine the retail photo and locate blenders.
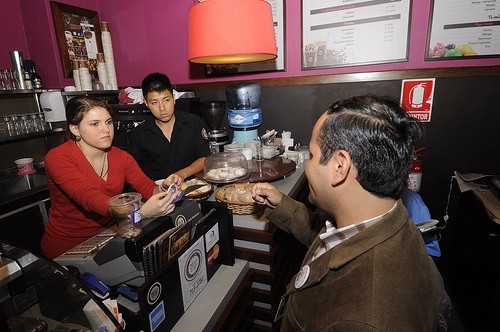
[200,101,229,152]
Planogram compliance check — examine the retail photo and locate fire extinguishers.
[406,146,432,193]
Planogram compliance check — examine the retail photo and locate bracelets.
[161,183,166,192]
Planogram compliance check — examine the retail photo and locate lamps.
[187,0,278,64]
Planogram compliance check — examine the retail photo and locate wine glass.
[0,111,52,138]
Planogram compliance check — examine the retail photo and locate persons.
[134,72,224,180]
[251,94,454,332]
[40,96,184,260]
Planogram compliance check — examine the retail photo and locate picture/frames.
[50,0,103,78]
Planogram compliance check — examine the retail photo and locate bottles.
[226,82,263,131]
[24,72,33,90]
[30,64,42,89]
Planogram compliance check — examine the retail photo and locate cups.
[72,20,119,91]
[0,69,21,90]
[224,141,260,163]
[14,158,34,174]
[107,193,142,238]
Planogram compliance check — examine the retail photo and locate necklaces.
[101,151,106,177]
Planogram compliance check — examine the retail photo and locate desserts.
[206,166,246,180]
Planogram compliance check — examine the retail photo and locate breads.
[180,182,210,195]
[217,183,255,203]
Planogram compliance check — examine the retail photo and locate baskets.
[215,182,272,215]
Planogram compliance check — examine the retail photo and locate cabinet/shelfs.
[0,89,50,145]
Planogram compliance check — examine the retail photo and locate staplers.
[79,272,110,300]
